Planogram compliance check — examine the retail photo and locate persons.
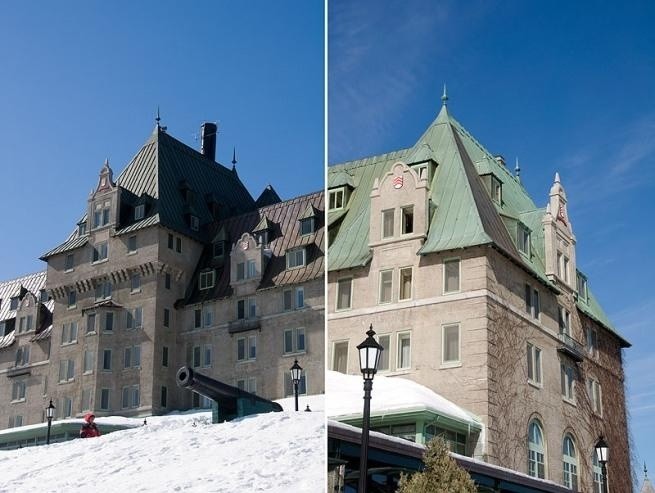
[80,412,101,437]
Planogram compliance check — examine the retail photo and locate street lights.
[45,397,56,442]
[355,322,383,492]
[288,358,303,410]
[596,433,611,491]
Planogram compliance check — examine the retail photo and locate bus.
[327,419,572,492]
